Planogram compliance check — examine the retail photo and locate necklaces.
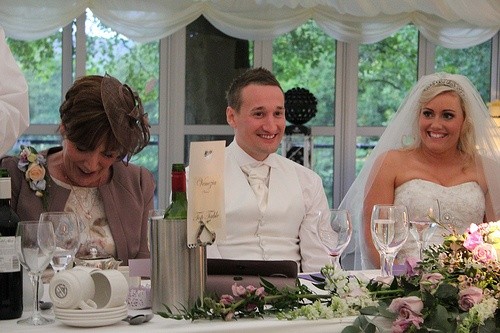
[62,151,102,219]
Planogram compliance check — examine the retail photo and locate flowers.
[17,145,46,196]
[158,222,500,333]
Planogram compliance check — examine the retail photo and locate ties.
[240,164,270,215]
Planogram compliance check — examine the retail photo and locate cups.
[37,211,81,273]
[145,209,166,254]
[49,269,97,310]
[90,267,130,310]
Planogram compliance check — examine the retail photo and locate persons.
[0,22,30,161]
[168,66,343,273]
[0,71,155,266]
[334,72,500,272]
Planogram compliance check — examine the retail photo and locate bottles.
[0,169,23,321]
[163,163,188,220]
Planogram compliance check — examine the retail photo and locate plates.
[54,301,129,327]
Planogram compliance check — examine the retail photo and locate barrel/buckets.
[150,217,205,317]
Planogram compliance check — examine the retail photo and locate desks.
[0,269,395,333]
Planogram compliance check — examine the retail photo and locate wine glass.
[370,203,409,278]
[14,219,57,327]
[316,208,353,271]
[374,219,396,278]
[408,195,440,261]
